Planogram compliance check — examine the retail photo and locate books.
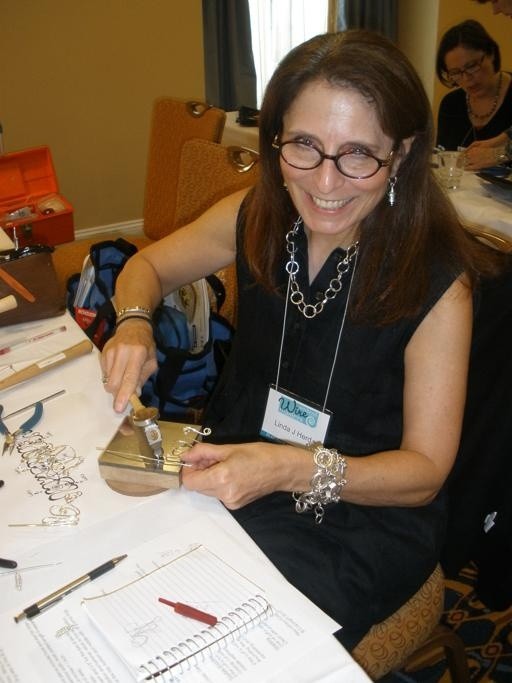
[95,409,206,489]
[168,278,213,352]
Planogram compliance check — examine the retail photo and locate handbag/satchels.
[0,246,65,328]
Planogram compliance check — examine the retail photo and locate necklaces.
[464,70,501,120]
[284,217,361,319]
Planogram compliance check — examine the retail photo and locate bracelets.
[495,136,506,163]
[113,314,154,328]
[290,441,347,523]
[116,305,152,318]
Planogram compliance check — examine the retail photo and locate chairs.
[354,223,512,683]
[50,97,229,302]
[127,136,264,297]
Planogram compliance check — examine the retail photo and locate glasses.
[446,53,485,82]
[272,135,401,180]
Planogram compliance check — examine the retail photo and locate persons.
[456,121,512,169]
[454,0,512,171]
[98,28,474,658]
[434,20,512,161]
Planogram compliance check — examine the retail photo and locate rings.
[464,157,468,163]
[464,154,468,158]
[100,376,111,384]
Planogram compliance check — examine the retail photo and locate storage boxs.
[0,144,76,248]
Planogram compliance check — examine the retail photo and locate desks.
[431,151,512,238]
[1,317,334,683]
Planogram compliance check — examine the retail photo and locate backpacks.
[67,238,234,422]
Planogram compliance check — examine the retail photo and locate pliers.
[0,402,43,456]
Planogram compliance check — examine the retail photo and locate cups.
[437,150,466,189]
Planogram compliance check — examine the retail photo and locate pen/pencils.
[0,326,66,355]
[13,554,128,624]
